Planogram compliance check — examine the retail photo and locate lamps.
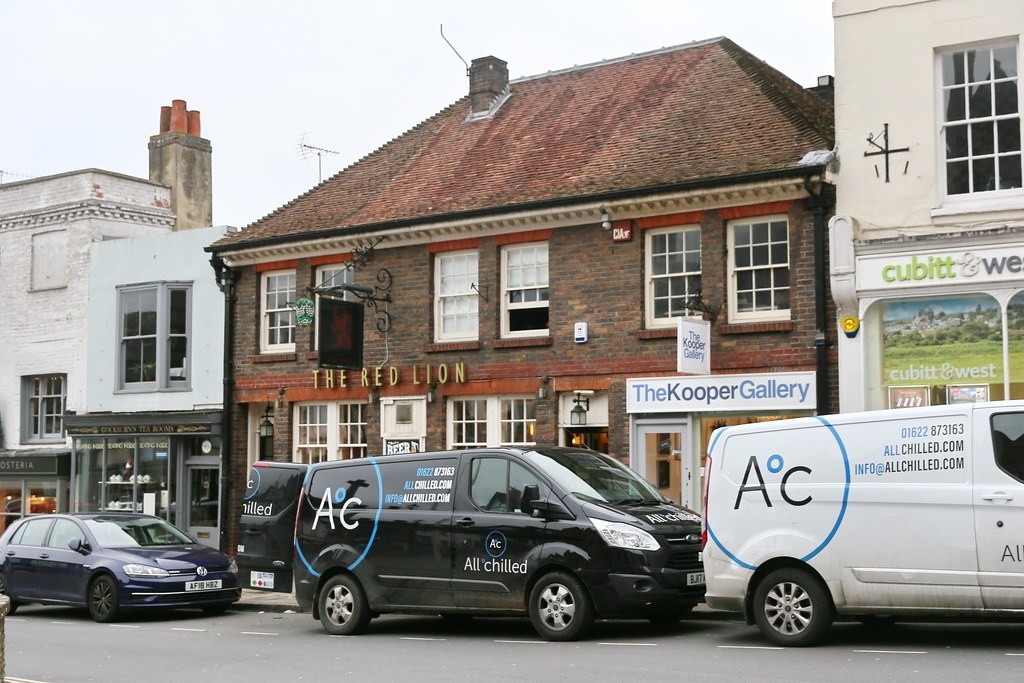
[276,387,287,408]
[539,388,547,398]
[259,397,275,437]
[369,386,379,408]
[427,382,439,403]
[571,393,589,425]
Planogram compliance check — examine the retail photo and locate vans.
[701,399,1024,649]
[236,442,707,642]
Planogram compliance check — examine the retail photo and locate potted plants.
[128,360,156,381]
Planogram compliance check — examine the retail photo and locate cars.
[0,510,243,624]
